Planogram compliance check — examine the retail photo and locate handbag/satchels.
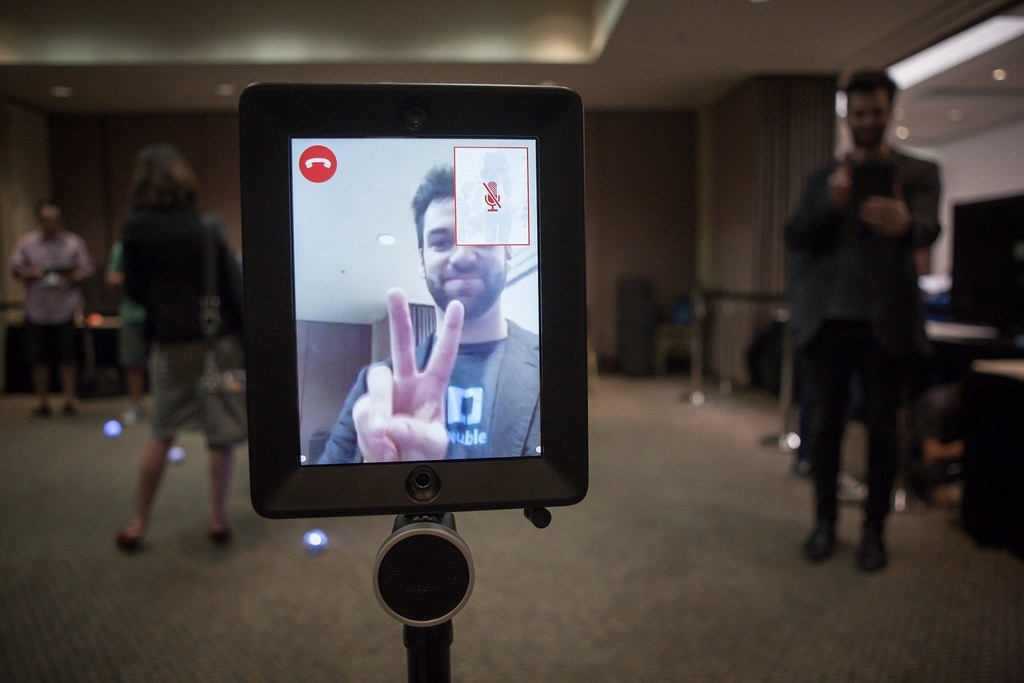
[199,368,248,447]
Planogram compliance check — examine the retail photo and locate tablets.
[848,162,897,208]
[238,82,589,520]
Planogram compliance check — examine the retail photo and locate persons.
[785,65,943,572]
[105,244,151,426]
[317,166,540,466]
[12,200,95,419]
[115,145,245,551]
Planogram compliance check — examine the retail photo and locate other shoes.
[208,528,230,544]
[65,406,74,417]
[809,518,835,558]
[33,406,49,420]
[117,527,138,550]
[858,527,885,571]
